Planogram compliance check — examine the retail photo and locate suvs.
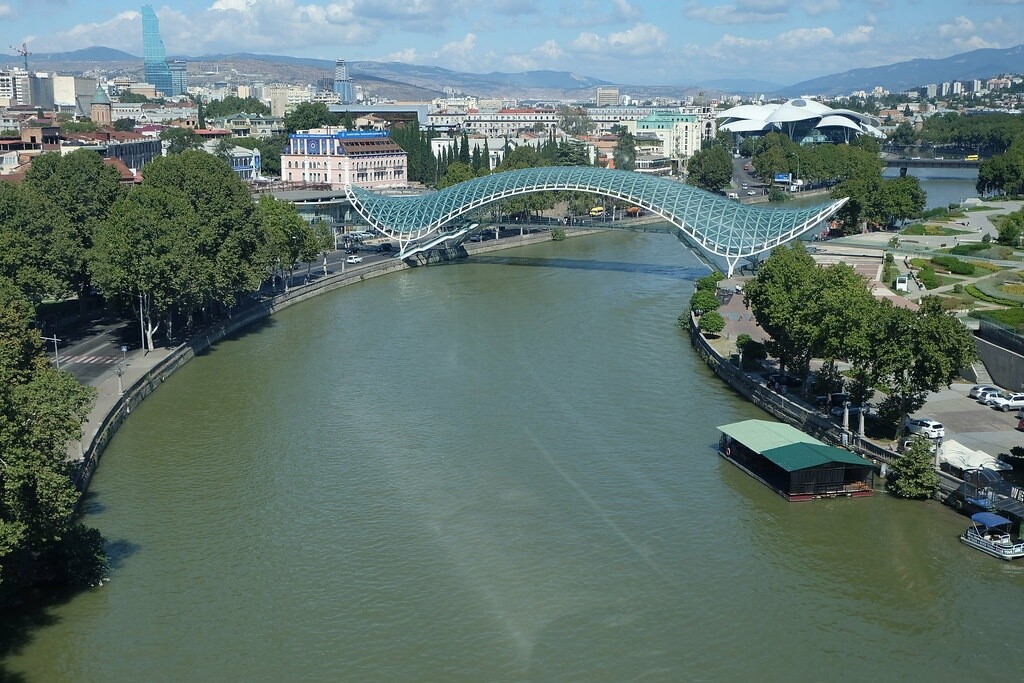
[994,392,1024,412]
[906,418,944,439]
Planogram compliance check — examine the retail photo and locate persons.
[767,379,787,397]
[812,218,837,242]
[735,283,745,295]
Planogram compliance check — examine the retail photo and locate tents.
[927,438,1015,486]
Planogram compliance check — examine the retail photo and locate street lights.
[792,152,799,180]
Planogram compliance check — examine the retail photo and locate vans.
[965,155,978,160]
[589,207,604,216]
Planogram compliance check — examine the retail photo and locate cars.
[816,393,870,417]
[742,181,747,189]
[347,255,363,264]
[970,385,1007,406]
[1017,407,1024,431]
[748,189,756,196]
[376,243,392,252]
[342,230,396,254]
[770,375,803,387]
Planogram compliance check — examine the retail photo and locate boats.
[715,419,876,502]
[960,513,1024,561]
